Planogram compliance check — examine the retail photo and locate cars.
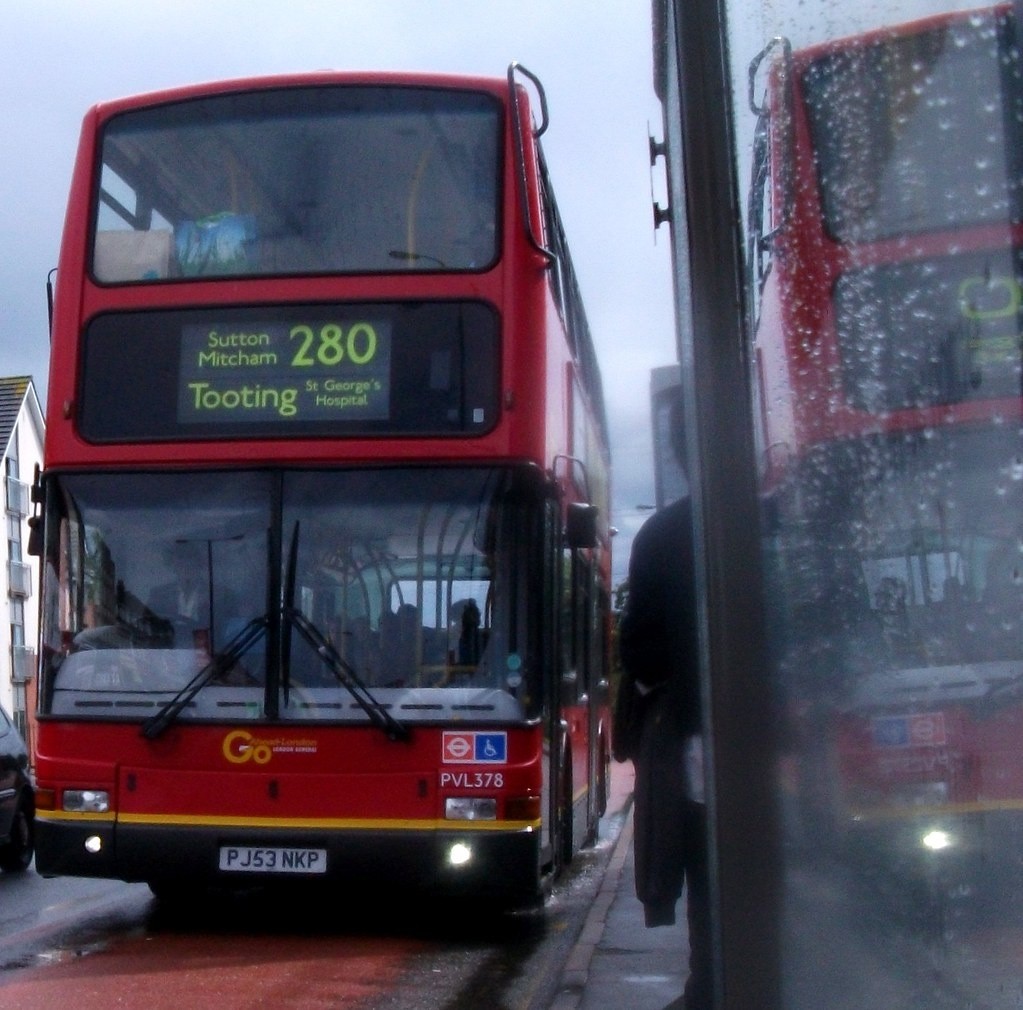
[0,705,33,874]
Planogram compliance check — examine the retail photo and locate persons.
[611,394,716,1010]
[144,538,239,654]
[315,598,493,689]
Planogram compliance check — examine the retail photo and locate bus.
[744,3,1023,919]
[23,60,614,915]
